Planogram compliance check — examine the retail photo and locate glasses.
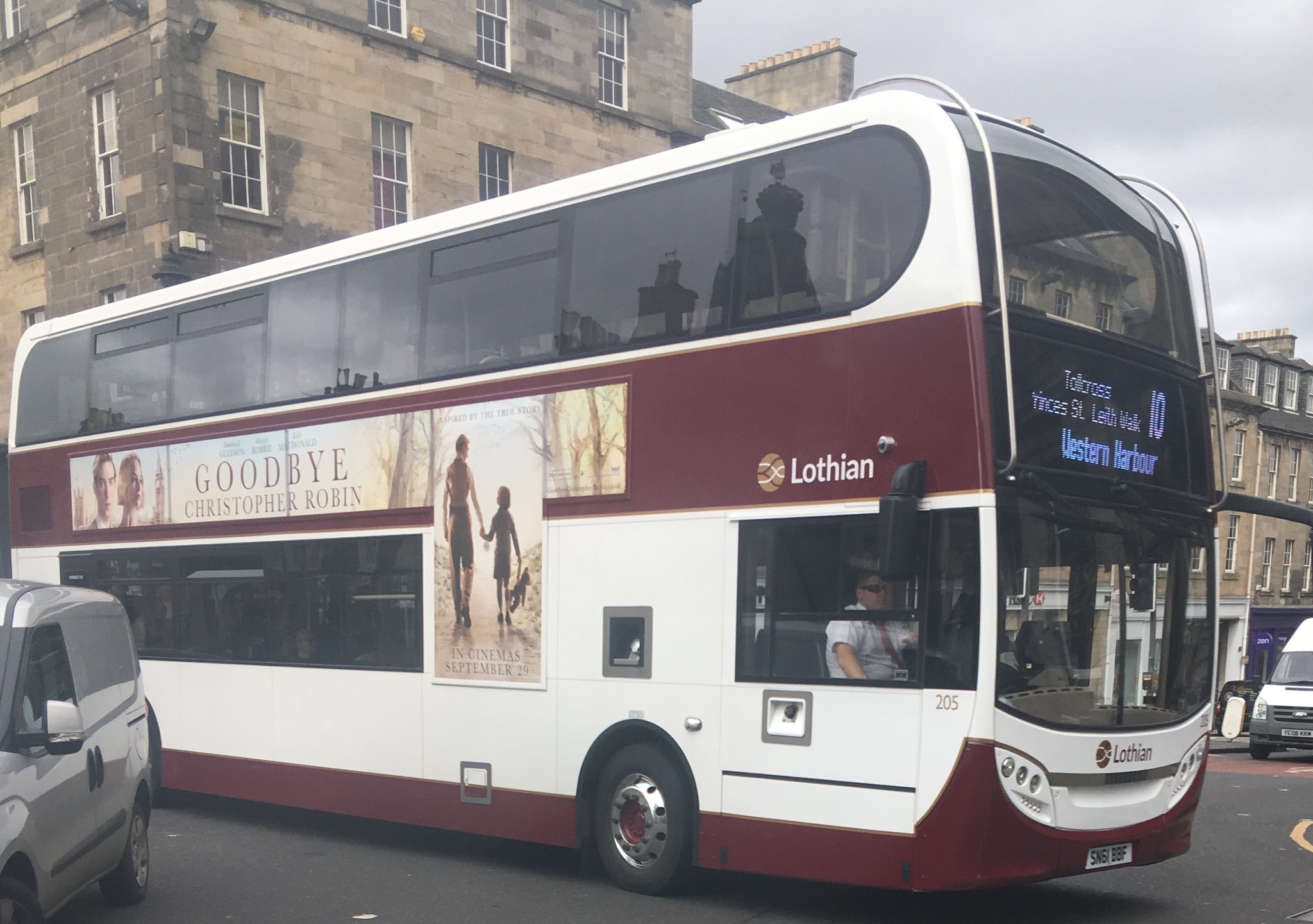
[858,584,890,594]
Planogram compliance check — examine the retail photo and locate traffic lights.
[1005,568,1027,599]
[1129,564,1157,613]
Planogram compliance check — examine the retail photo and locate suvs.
[0,576,155,924]
[1214,679,1267,737]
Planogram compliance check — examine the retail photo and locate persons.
[133,616,149,648]
[442,434,522,628]
[295,629,315,659]
[824,568,919,679]
[86,452,145,530]
[942,558,1052,691]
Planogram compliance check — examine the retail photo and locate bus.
[4,74,1230,899]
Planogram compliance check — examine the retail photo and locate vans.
[1247,617,1313,761]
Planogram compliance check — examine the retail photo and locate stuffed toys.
[510,563,532,613]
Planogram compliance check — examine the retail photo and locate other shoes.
[457,618,463,624]
[506,611,511,625]
[497,613,505,626]
[461,605,473,628]
